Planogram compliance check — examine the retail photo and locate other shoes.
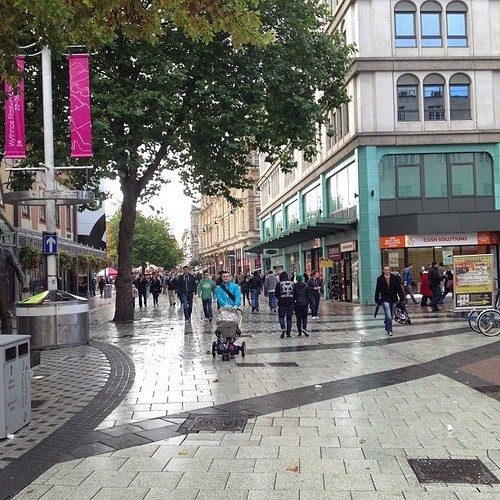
[243,304,245,306]
[256,309,259,311]
[274,310,276,312]
[140,305,142,308]
[252,307,255,312]
[157,303,159,306]
[249,301,250,306]
[145,304,147,307]
[271,308,272,310]
[173,302,176,305]
[153,305,156,308]
[170,303,172,306]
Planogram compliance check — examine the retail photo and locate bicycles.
[476,309,500,336]
[469,309,494,334]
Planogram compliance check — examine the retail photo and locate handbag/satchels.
[395,300,411,324]
[241,281,247,292]
[149,279,153,293]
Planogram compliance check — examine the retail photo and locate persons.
[90,271,179,307]
[428,262,448,311]
[198,272,216,322]
[421,261,454,306]
[193,266,249,301]
[247,271,263,312]
[215,271,241,309]
[401,263,420,305]
[240,276,251,306]
[307,270,324,319]
[178,266,197,322]
[275,271,295,338]
[264,270,279,312]
[136,274,149,308]
[293,273,311,338]
[375,264,404,336]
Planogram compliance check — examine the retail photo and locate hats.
[296,274,303,281]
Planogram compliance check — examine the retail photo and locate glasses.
[223,276,229,278]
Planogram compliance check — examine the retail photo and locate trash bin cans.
[2,335,33,440]
[104,284,114,298]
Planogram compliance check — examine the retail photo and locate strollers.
[213,306,247,361]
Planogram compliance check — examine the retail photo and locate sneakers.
[297,335,302,338]
[389,332,393,336]
[209,317,212,322]
[280,329,286,338]
[185,319,189,324]
[302,329,309,337]
[415,301,420,304]
[434,307,441,311]
[287,335,291,339]
[316,316,319,319]
[203,318,209,322]
[312,316,316,319]
[189,318,191,322]
[429,303,436,312]
